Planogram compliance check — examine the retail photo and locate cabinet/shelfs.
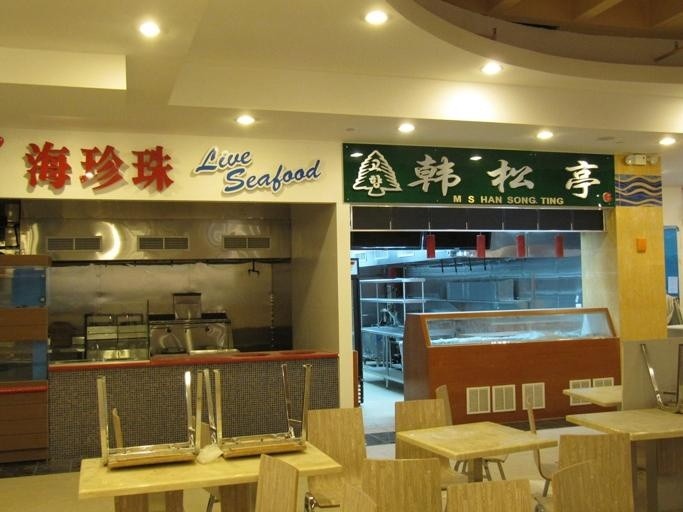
[404,267,581,338]
[359,277,427,388]
[0,253,52,474]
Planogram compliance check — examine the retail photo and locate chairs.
[97,370,205,512]
[204,363,312,512]
[306,396,534,511]
[532,458,621,510]
[254,453,307,512]
[526,398,560,495]
[558,431,634,511]
[640,343,683,413]
